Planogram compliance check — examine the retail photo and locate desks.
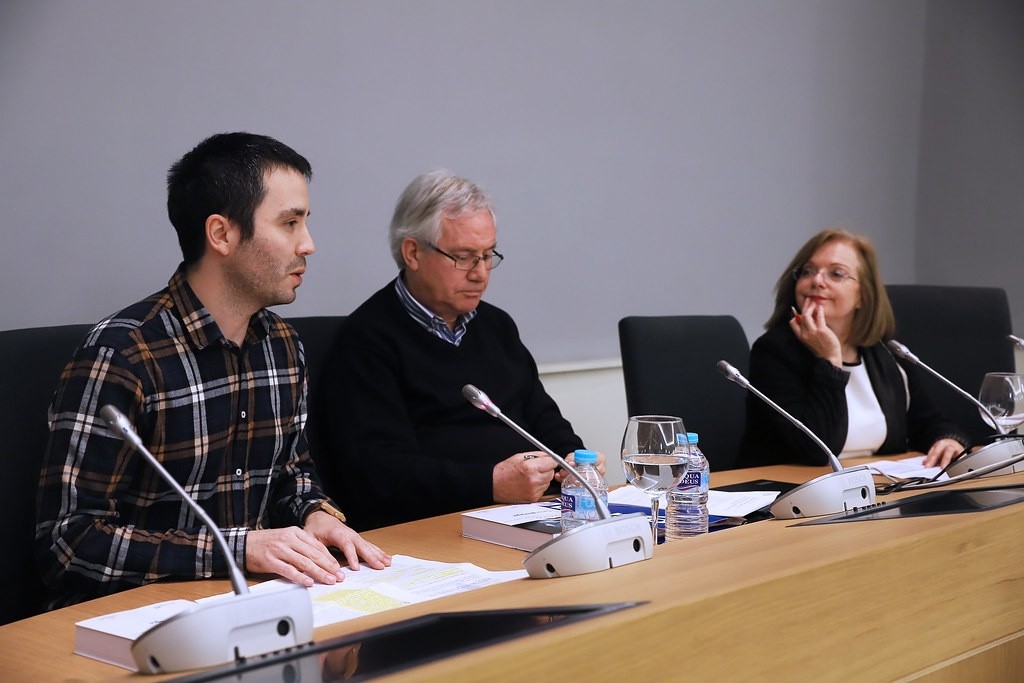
[0,443,1023,683]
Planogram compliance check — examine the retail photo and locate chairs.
[882,284,1016,443]
[1,324,95,628]
[619,315,751,475]
[282,316,347,463]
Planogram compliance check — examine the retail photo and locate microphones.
[99,403,314,674]
[886,335,1024,478]
[462,384,655,578]
[717,360,876,519]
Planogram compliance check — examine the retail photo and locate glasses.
[794,264,859,283]
[423,239,503,271]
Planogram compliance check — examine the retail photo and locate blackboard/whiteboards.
[0,0,1023,375]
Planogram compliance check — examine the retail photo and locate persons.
[314,170,606,534]
[743,229,971,469]
[33,132,392,611]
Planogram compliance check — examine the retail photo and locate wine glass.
[620,415,690,545]
[978,372,1024,442]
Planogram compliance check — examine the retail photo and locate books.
[73,600,197,673]
[460,502,561,553]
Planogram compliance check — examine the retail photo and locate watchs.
[301,499,346,530]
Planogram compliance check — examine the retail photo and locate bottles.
[666,433,708,542]
[561,450,608,534]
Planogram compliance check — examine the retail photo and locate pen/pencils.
[790,305,801,325]
[524,454,539,459]
